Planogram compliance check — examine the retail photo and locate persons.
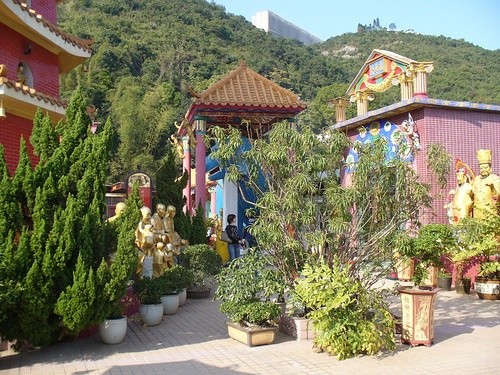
[134,202,189,280]
[17,63,30,86]
[104,201,126,223]
[443,171,473,226]
[224,214,245,270]
[466,149,500,221]
[170,134,183,157]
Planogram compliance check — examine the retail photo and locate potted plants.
[131,275,179,326]
[178,242,224,299]
[98,300,132,346]
[397,223,454,349]
[392,229,418,282]
[155,267,187,315]
[165,264,195,308]
[456,258,477,295]
[435,266,453,290]
[453,230,500,300]
[185,109,453,344]
[210,243,287,348]
